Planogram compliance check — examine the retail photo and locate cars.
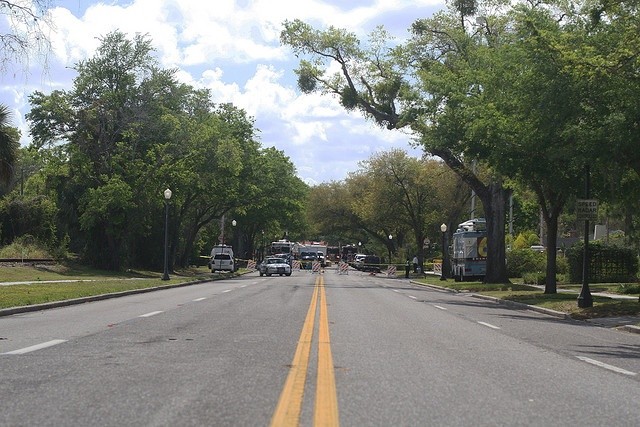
[342,245,380,273]
[259,258,292,277]
[212,252,234,273]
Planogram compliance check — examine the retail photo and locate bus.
[270,241,292,258]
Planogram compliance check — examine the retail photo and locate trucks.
[326,246,341,264]
[448,218,507,282]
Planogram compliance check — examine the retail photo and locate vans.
[317,249,325,263]
[209,244,234,269]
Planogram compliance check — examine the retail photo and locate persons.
[412,256,419,272]
[405,258,410,277]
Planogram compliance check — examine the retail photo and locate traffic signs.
[576,199,598,222]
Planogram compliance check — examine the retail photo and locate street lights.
[440,223,448,281]
[219,218,237,277]
[160,188,173,281]
[388,235,393,265]
